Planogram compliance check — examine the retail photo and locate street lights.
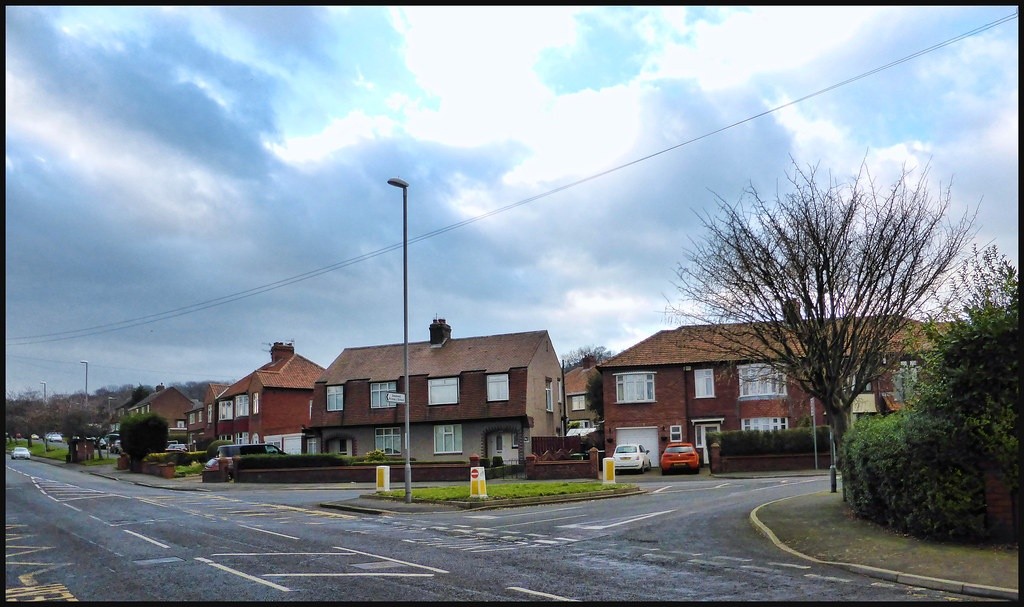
[80,360,89,462]
[40,381,49,452]
[107,397,118,457]
[386,174,413,505]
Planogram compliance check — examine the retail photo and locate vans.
[216,442,289,458]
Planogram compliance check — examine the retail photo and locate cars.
[569,444,605,466]
[203,456,235,481]
[165,443,189,452]
[16,432,63,442]
[109,438,122,455]
[90,437,107,450]
[659,442,701,475]
[10,447,32,460]
[611,444,652,476]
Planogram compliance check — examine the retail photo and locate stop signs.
[470,469,479,479]
